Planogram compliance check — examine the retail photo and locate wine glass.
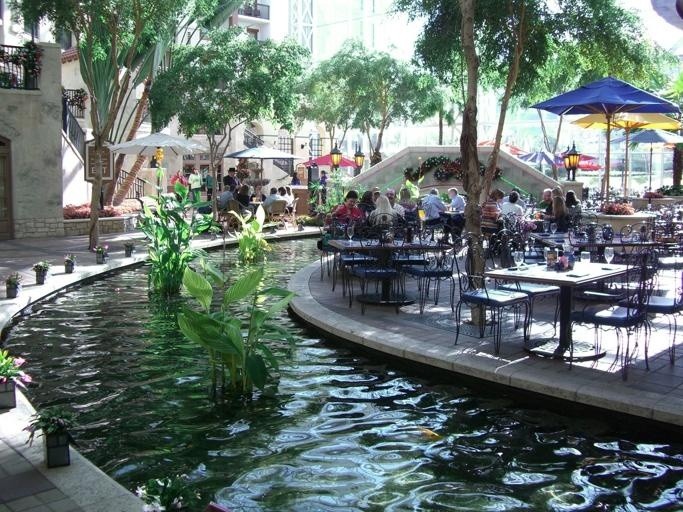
[346,227,353,242]
[512,222,615,273]
[647,204,651,212]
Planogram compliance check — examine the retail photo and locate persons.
[321,170,326,204]
[292,172,300,185]
[170,168,292,226]
[324,186,580,234]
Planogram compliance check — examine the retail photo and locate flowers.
[0,350,31,390]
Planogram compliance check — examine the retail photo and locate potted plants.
[6,272,21,298]
[124,241,134,257]
[31,260,50,284]
[64,253,77,274]
[93,244,108,264]
[24,407,73,468]
[296,217,305,231]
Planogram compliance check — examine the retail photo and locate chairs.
[452,193,681,383]
[218,197,299,237]
[318,192,469,315]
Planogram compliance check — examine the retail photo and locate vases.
[0,383,16,408]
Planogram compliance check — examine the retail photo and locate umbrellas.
[521,147,563,169]
[610,128,683,191]
[529,70,683,202]
[570,112,683,195]
[224,147,301,201]
[553,153,596,162]
[479,140,524,155]
[108,133,208,196]
[304,152,357,169]
[557,162,600,170]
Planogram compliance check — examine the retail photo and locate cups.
[252,197,255,202]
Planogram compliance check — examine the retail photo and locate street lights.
[566,141,581,181]
[559,145,574,182]
[353,144,365,175]
[331,140,341,178]
